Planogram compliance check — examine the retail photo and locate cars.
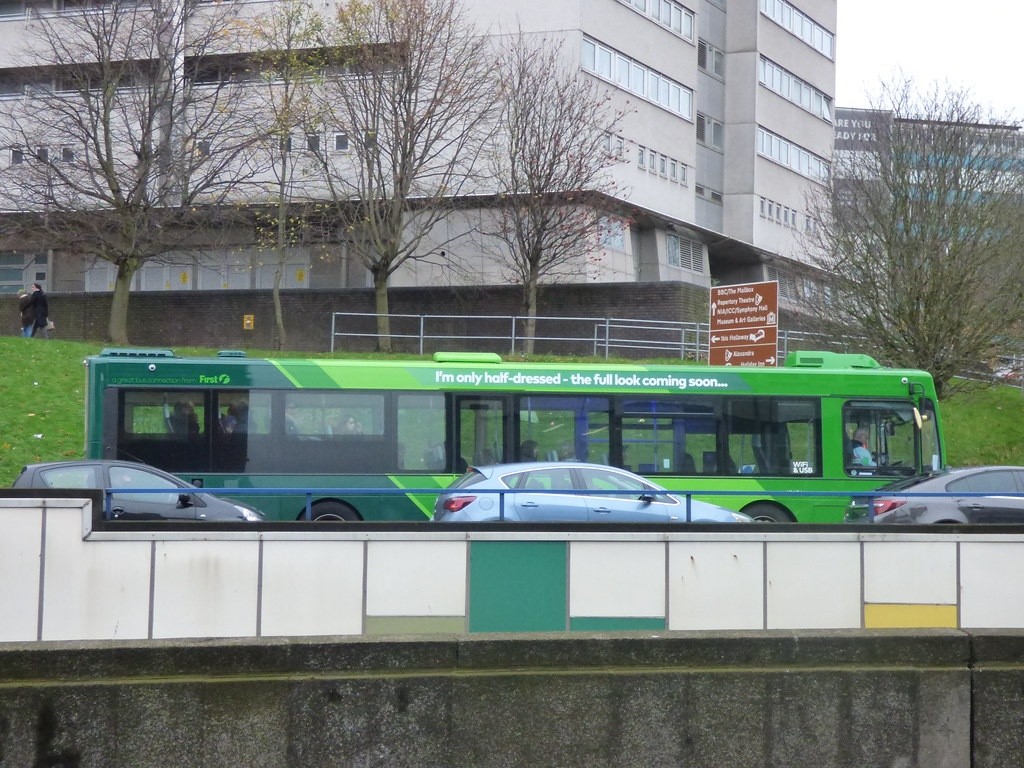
[993,364,1024,388]
[427,460,757,527]
[842,464,1024,534]
[14,458,269,524]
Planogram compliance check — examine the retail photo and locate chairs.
[752,433,772,475]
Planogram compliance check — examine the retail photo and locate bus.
[82,347,949,518]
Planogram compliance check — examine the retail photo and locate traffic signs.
[706,281,777,366]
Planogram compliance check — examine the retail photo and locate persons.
[849,427,878,472]
[31,283,49,339]
[220,402,255,435]
[336,414,364,435]
[172,400,199,433]
[520,439,539,462]
[17,289,36,338]
